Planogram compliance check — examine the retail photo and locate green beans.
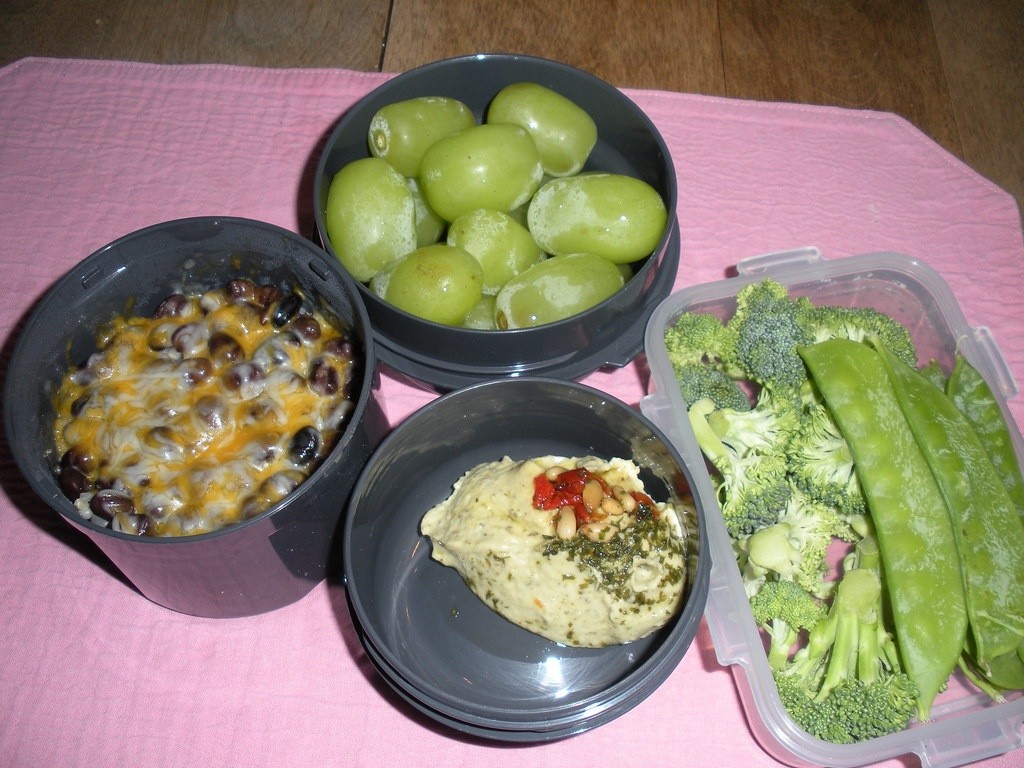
[796,332,1024,720]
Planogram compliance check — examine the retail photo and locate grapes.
[325,80,670,330]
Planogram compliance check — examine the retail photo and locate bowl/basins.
[637,243,1024,767]
[311,53,681,394]
[342,377,712,744]
[0,216,395,621]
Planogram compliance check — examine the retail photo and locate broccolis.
[662,281,921,744]
